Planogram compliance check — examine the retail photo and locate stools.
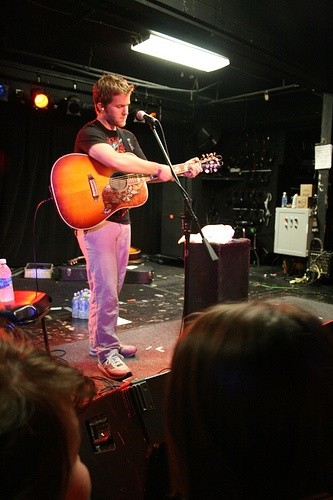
[0,290,53,357]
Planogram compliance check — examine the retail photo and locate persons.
[164,301,333,500]
[73,74,203,380]
[0,329,97,500]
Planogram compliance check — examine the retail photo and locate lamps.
[0,73,97,121]
[128,26,230,72]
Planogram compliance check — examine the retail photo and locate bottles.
[282,192,287,207]
[72,289,91,319]
[291,193,298,208]
[0,259,14,302]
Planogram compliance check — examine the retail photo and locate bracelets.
[157,166,162,176]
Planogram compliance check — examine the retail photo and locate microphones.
[136,110,158,124]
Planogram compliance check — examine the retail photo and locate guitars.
[49,151,220,230]
[67,246,142,265]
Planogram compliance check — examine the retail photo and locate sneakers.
[90,344,136,357]
[95,354,132,381]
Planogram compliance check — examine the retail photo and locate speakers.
[265,294,333,327]
[77,368,175,500]
[184,237,251,323]
